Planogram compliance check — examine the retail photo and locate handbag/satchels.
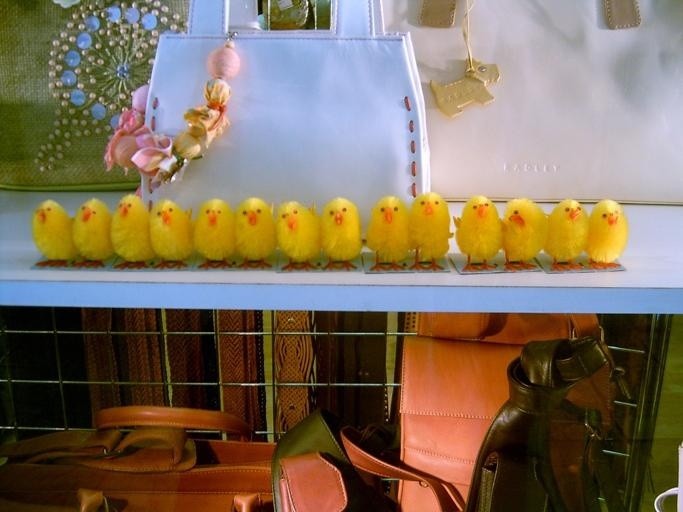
[1,406,465,512]
[400,312,629,508]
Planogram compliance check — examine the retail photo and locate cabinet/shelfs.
[0,170,682,315]
